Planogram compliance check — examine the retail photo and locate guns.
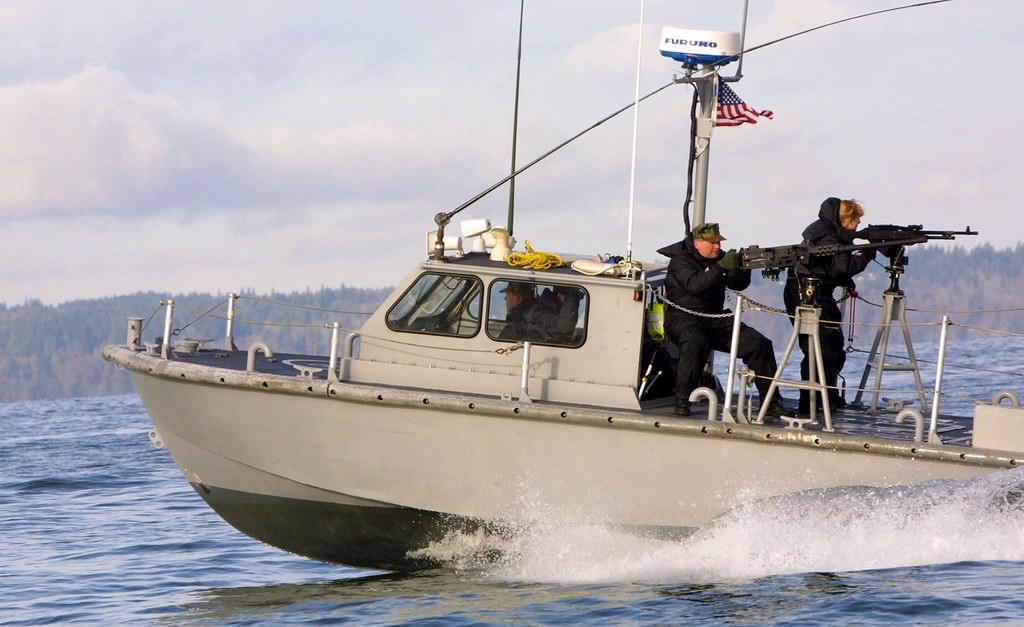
[737,231,955,270]
[855,222,980,240]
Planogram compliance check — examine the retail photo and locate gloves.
[717,248,740,271]
[565,287,584,308]
[856,247,881,261]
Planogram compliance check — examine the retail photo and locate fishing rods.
[435,0,952,225]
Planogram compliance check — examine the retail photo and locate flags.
[712,74,774,127]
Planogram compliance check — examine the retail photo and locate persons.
[783,198,878,414]
[656,223,795,419]
[496,281,584,345]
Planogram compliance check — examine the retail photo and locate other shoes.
[815,396,847,415]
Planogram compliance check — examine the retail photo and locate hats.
[499,281,539,294]
[692,222,727,244]
[553,286,573,291]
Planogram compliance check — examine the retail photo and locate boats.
[100,0,1024,580]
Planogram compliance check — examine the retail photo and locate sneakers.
[759,402,795,418]
[674,397,691,416]
